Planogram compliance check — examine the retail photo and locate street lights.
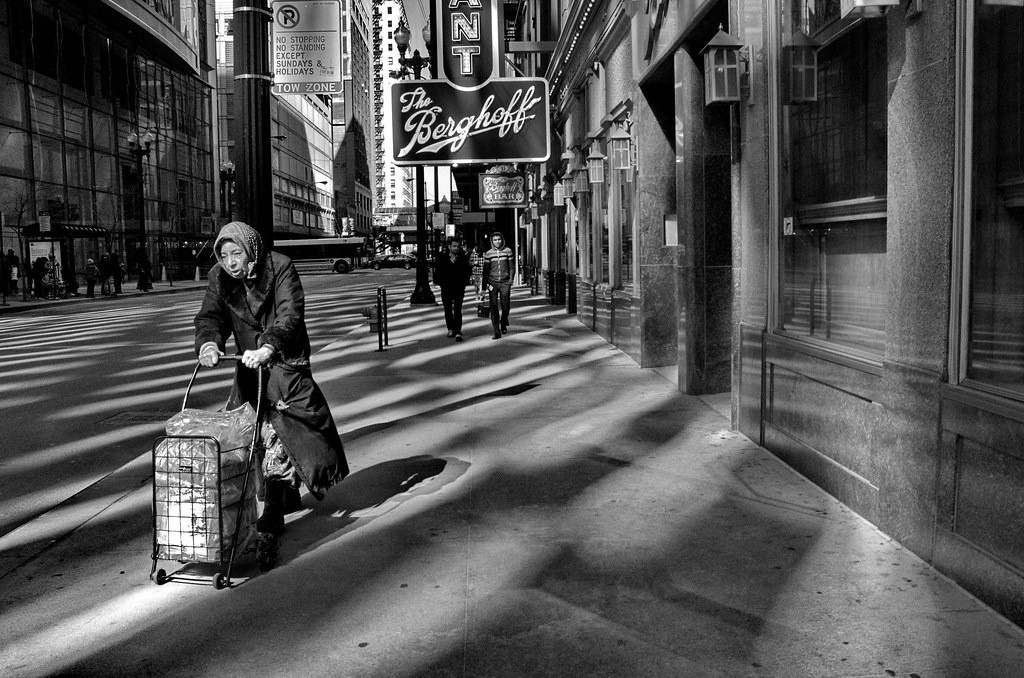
[308,181,327,236]
[394,14,436,306]
[407,178,429,272]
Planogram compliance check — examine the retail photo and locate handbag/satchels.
[11,267,18,280]
[477,295,490,318]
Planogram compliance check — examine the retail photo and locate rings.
[250,359,254,361]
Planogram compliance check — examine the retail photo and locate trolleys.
[149,354,277,590]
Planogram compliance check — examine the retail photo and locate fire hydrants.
[361,306,384,332]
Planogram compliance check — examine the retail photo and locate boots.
[254,477,285,533]
[280,479,301,515]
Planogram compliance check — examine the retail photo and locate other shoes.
[455,335,463,343]
[492,333,502,339]
[447,330,454,337]
[501,325,507,334]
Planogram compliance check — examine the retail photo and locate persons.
[0,248,155,299]
[433,232,515,341]
[192,222,349,534]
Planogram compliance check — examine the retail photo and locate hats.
[87,259,94,264]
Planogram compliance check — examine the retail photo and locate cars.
[367,254,417,270]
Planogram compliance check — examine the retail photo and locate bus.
[274,237,368,273]
